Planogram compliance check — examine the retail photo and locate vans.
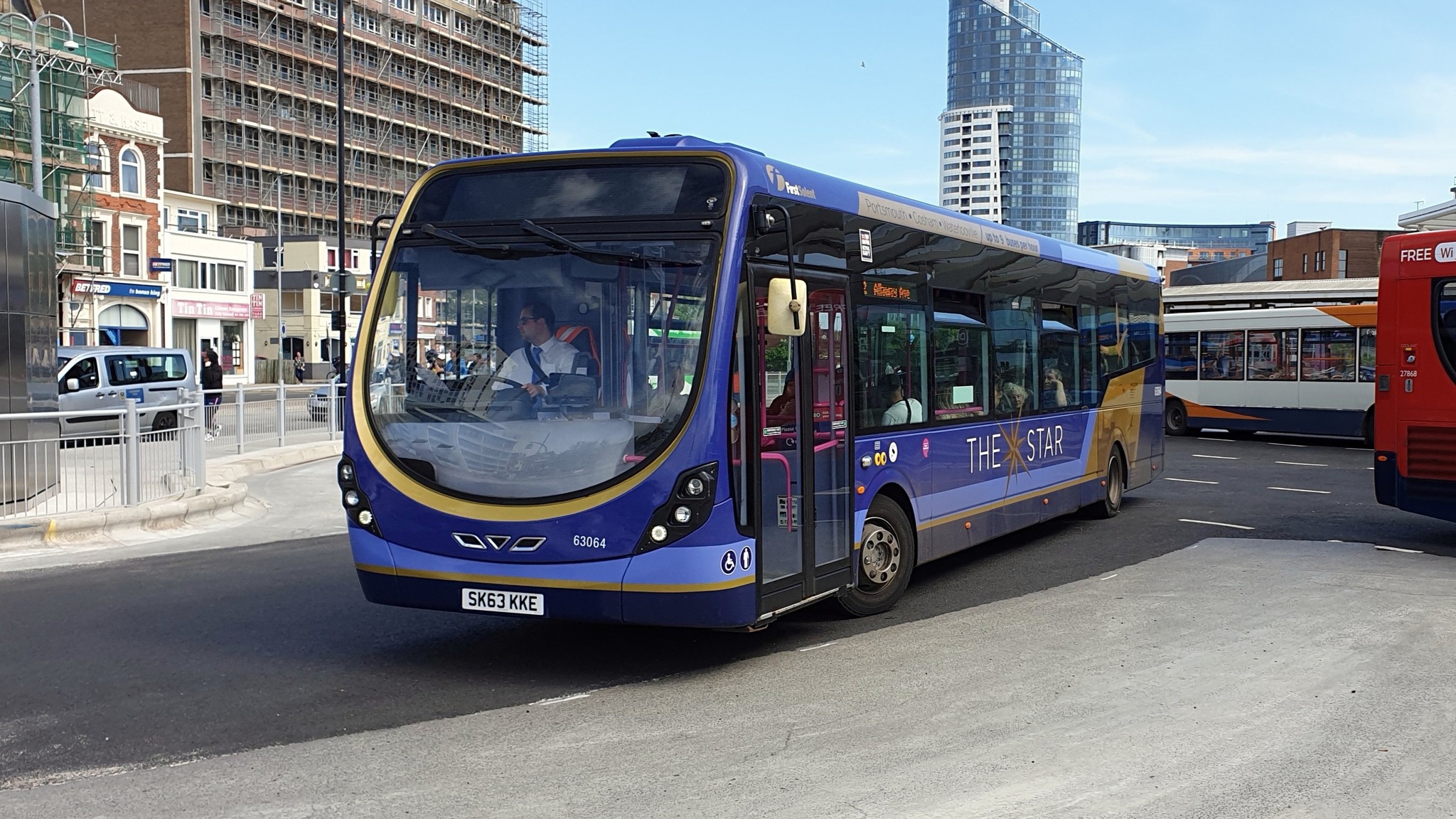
[57,345,196,442]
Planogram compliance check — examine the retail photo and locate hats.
[438,354,446,360]
[391,350,400,357]
[201,351,208,358]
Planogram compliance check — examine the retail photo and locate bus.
[1163,305,1377,449]
[334,132,1167,641]
[1375,230,1455,525]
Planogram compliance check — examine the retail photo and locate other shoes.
[300,382,303,385]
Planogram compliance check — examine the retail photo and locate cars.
[307,368,387,422]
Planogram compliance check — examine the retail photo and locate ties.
[531,347,542,409]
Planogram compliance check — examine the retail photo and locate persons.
[385,350,405,384]
[1042,365,1071,408]
[199,350,223,442]
[65,361,89,388]
[1330,362,1348,378]
[293,351,305,384]
[143,359,152,380]
[766,369,796,426]
[885,363,894,375]
[468,353,487,376]
[492,301,587,398]
[444,350,467,375]
[1180,348,1236,377]
[648,365,689,421]
[880,374,922,426]
[430,354,446,377]
[994,375,1028,414]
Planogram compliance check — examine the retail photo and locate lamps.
[269,272,276,277]
[306,341,310,347]
[345,342,347,347]
[315,341,318,347]
[265,340,267,346]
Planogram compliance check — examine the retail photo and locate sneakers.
[212,425,222,437]
[204,435,214,441]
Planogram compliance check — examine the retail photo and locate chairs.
[556,324,602,381]
[1440,308,1456,373]
[1165,353,1344,379]
[1003,339,1138,413]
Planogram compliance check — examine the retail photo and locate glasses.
[518,317,547,325]
